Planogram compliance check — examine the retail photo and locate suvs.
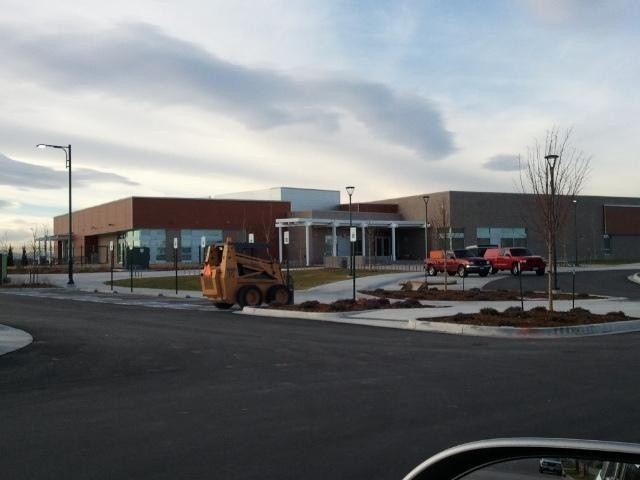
[482,246,546,277]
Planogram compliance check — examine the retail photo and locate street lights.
[36,143,77,286]
[544,153,563,292]
[345,185,357,276]
[422,194,432,276]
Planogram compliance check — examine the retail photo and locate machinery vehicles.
[201,239,293,310]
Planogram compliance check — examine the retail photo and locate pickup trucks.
[423,246,493,278]
